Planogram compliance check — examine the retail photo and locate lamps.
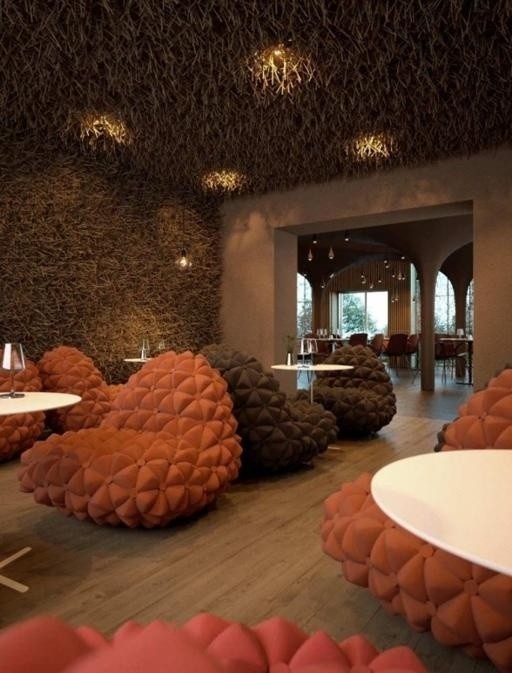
[173,197,191,270]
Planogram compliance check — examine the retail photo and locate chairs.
[298,342,398,438]
[318,366,512,672]
[19,352,240,528]
[300,327,475,388]
[37,346,125,433]
[0,350,43,465]
[200,345,338,477]
[1,602,429,671]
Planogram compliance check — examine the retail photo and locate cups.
[143,338,149,353]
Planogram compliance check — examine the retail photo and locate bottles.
[140,349,146,359]
[285,351,294,365]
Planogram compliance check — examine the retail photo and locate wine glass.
[1,342,27,399]
[298,337,318,367]
[456,328,464,337]
[316,327,339,339]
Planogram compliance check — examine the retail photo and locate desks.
[1,389,82,592]
[124,357,154,363]
[368,443,512,590]
[271,364,354,451]
